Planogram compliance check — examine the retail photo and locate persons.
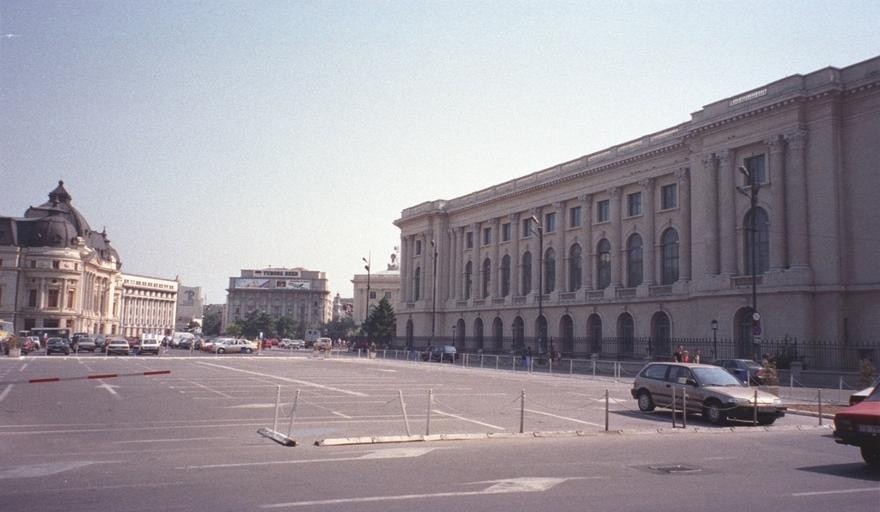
[673,344,700,363]
[762,353,776,369]
[163,334,169,353]
[190,336,204,353]
[346,339,376,359]
[521,343,532,368]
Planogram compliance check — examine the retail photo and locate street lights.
[711,319,718,361]
[362,257,370,324]
[452,326,456,345]
[735,165,758,362]
[512,324,516,354]
[430,239,438,345]
[531,215,542,366]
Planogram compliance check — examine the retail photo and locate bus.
[0,320,14,338]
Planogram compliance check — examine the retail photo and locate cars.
[631,358,788,426]
[421,345,459,363]
[833,375,880,467]
